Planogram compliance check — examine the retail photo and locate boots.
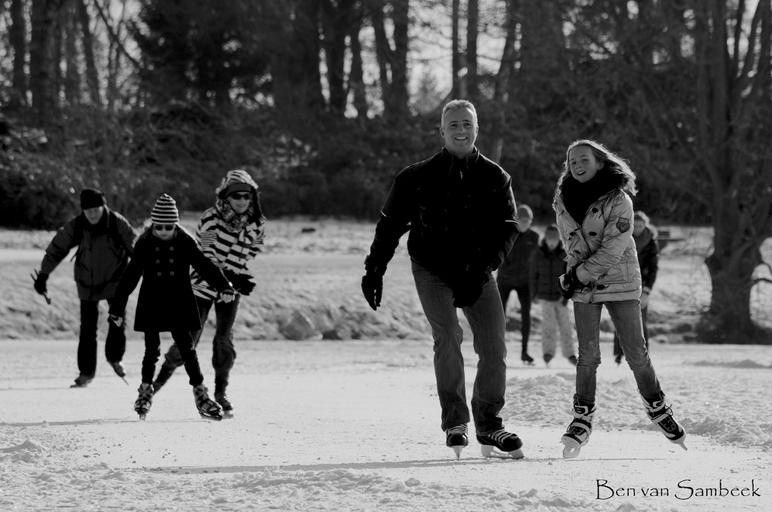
[135,383,154,414]
[638,392,686,442]
[561,395,596,446]
[193,384,219,414]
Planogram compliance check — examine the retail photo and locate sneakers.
[521,351,624,364]
[76,372,93,384]
[214,392,232,411]
[447,423,468,445]
[476,427,522,451]
[110,361,124,377]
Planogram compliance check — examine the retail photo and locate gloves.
[107,314,124,328]
[558,270,575,298]
[361,270,383,310]
[221,289,236,304]
[640,287,650,310]
[34,272,48,294]
[232,274,256,295]
[452,274,490,308]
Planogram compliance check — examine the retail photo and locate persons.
[32,185,140,386]
[106,189,237,415]
[548,136,688,448]
[525,221,582,365]
[614,209,660,355]
[360,97,524,452]
[150,170,268,412]
[494,204,540,363]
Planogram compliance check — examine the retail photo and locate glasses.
[155,225,174,231]
[230,192,250,200]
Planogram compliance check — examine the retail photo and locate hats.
[151,194,179,224]
[516,207,534,218]
[80,189,107,209]
[545,227,560,239]
